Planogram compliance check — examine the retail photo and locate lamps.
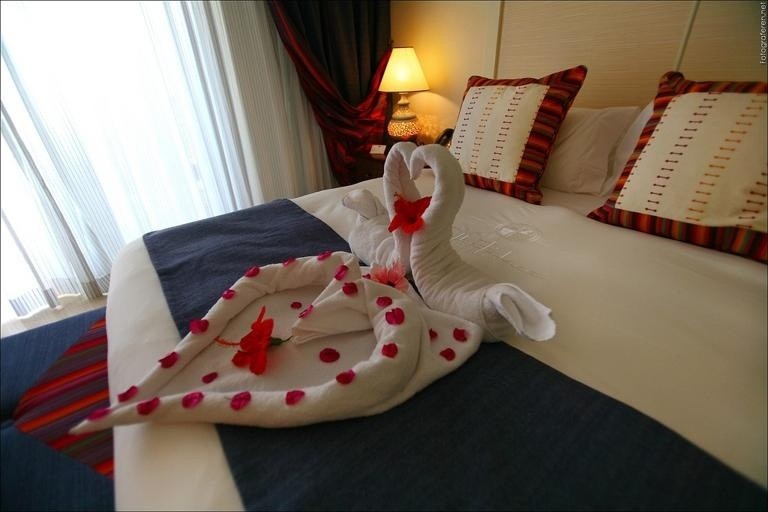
[378,45,430,142]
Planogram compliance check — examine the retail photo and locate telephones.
[436,128,454,145]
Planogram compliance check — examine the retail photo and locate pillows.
[444,63,766,264]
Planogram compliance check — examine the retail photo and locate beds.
[99,163,768,512]
